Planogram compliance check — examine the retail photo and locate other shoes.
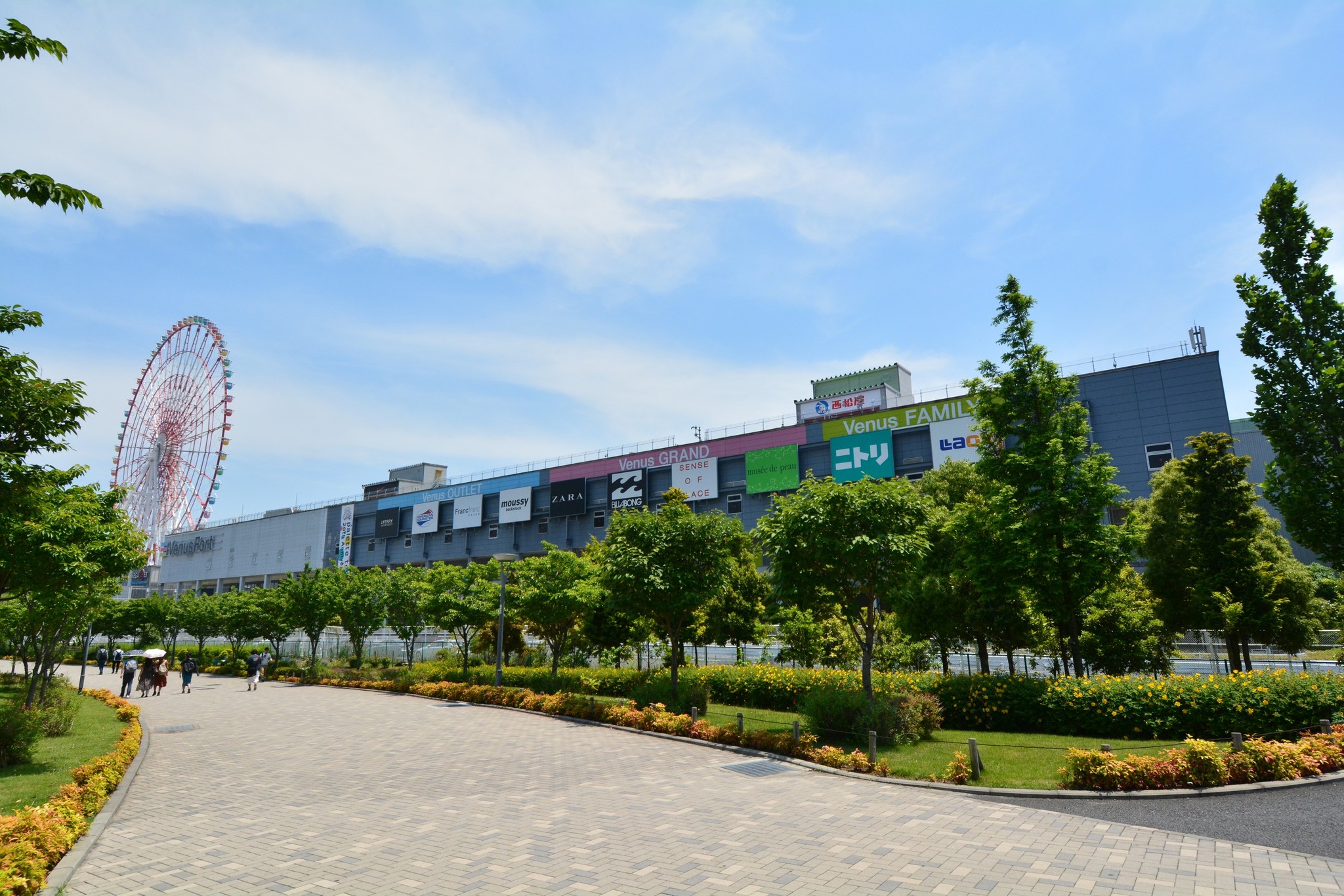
[261,678,264,682]
[182,691,185,693]
[248,687,251,691]
[152,691,156,696]
[126,695,129,698]
[119,694,123,698]
[254,684,257,691]
[187,688,190,694]
[157,692,160,696]
[141,694,144,697]
[145,695,147,697]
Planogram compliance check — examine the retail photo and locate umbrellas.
[120,649,146,657]
[141,648,166,659]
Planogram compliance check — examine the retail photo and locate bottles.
[126,664,129,669]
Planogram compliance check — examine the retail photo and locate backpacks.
[125,658,137,673]
[183,659,194,673]
[115,650,123,660]
[99,649,107,662]
[157,659,167,676]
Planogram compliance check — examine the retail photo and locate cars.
[366,638,411,659]
[414,638,473,660]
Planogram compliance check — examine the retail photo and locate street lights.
[492,552,518,687]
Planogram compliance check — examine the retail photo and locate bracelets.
[179,673,181,674]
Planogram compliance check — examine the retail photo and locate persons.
[96,645,108,675]
[212,655,227,666]
[179,652,198,693]
[259,647,273,682]
[135,657,154,698]
[246,649,262,691]
[152,656,169,696]
[112,645,124,674]
[118,656,138,698]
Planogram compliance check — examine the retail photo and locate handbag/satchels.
[246,668,256,678]
